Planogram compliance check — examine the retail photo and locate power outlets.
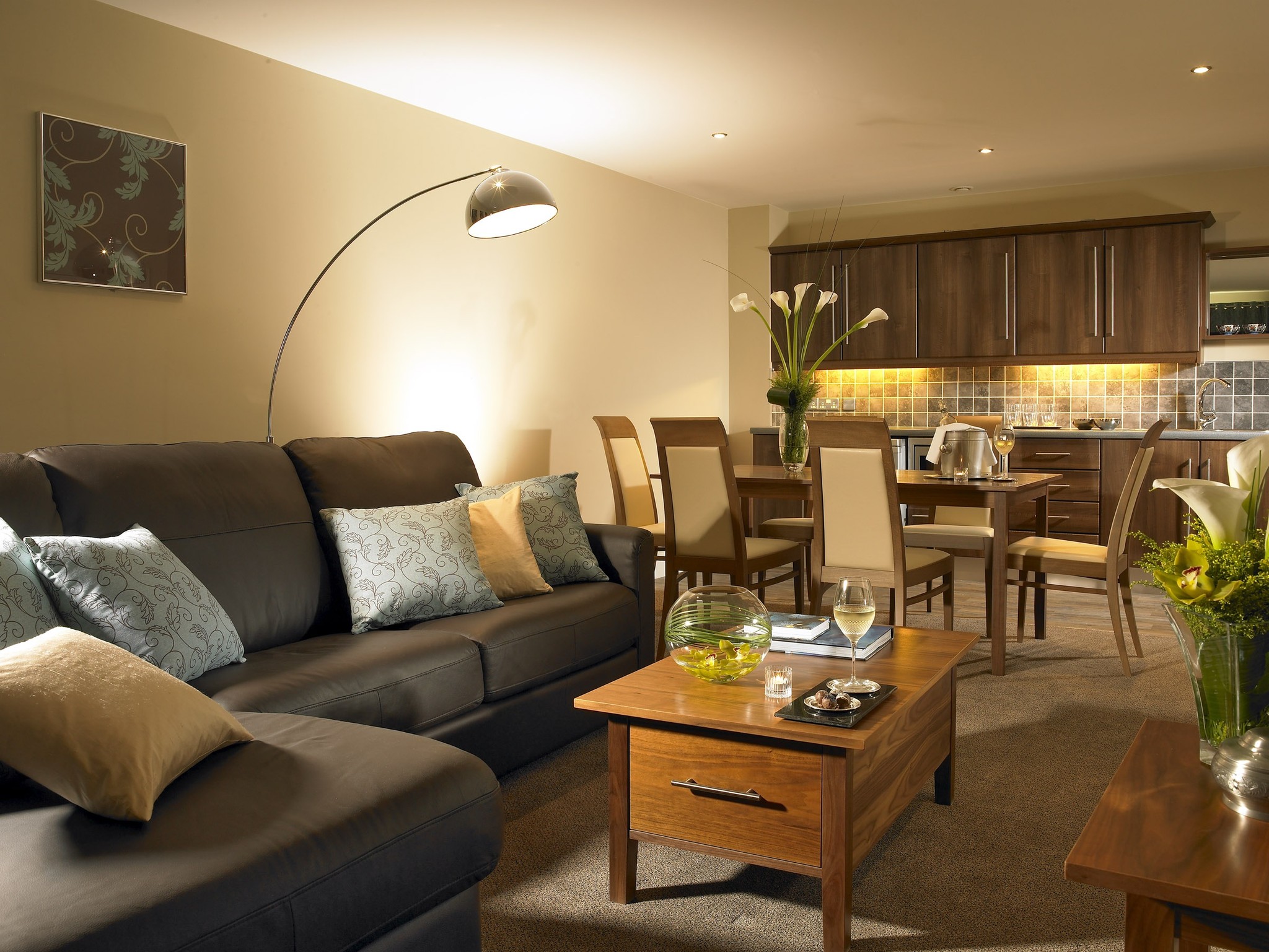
[818,398,839,410]
[806,398,819,410]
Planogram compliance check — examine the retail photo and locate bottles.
[938,400,958,426]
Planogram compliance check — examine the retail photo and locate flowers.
[1124,432,1269,640]
[701,194,889,415]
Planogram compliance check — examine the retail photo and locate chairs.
[649,416,804,663]
[758,500,813,606]
[901,413,1004,639]
[592,415,698,604]
[1006,417,1173,678]
[800,416,955,632]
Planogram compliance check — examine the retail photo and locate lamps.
[265,163,558,446]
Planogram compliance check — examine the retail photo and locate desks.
[647,462,1064,677]
[1062,717,1269,952]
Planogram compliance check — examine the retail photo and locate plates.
[826,678,881,694]
[849,690,881,700]
[803,705,861,719]
[804,695,861,711]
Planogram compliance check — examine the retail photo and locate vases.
[778,413,810,473]
[1162,600,1269,767]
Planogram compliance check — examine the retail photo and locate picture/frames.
[34,110,188,296]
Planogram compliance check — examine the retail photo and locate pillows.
[466,484,554,603]
[0,516,67,651]
[22,520,249,684]
[318,494,505,637]
[454,469,609,589]
[0,624,257,822]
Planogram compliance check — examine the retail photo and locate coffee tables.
[572,610,983,952]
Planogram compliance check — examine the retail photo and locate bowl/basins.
[1072,416,1121,431]
[1215,322,1266,334]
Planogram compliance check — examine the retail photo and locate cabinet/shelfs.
[752,434,813,544]
[1009,437,1100,546]
[767,211,1218,372]
[1100,439,1269,568]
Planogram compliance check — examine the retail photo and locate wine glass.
[834,576,876,689]
[993,424,1015,481]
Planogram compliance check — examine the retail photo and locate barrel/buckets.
[936,428,986,477]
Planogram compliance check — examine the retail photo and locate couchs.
[0,427,660,951]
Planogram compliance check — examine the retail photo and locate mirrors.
[1201,245,1269,340]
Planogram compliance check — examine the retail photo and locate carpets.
[476,613,1240,952]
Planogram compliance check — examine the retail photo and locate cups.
[954,467,969,483]
[1005,401,1058,427]
[764,665,794,699]
